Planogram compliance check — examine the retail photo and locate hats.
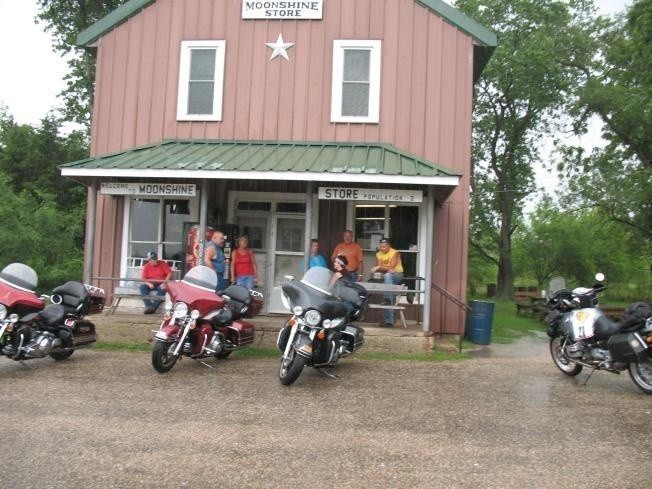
[379,237,392,244]
[147,251,157,261]
[335,255,348,267]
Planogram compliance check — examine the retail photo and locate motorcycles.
[540,273,652,395]
[0,262,106,360]
[276,266,367,386]
[152,265,263,373]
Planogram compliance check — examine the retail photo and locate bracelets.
[356,274,363,277]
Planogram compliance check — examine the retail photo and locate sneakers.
[378,322,393,328]
[379,298,391,306]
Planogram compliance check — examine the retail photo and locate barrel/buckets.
[467,300,494,345]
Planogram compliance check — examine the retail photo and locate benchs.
[102,266,169,316]
[354,273,423,327]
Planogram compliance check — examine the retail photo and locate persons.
[330,230,363,282]
[299,238,327,275]
[203,231,230,292]
[324,254,353,290]
[370,237,405,327]
[230,235,260,288]
[138,251,173,315]
